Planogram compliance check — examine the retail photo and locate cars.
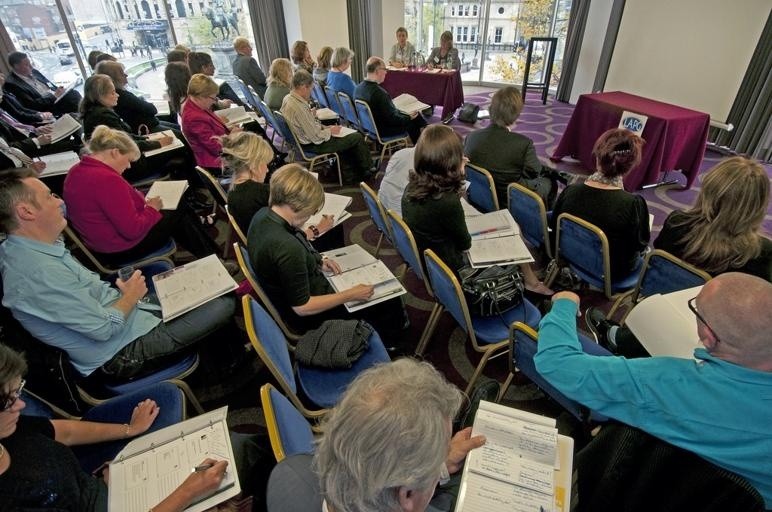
[58,55,72,66]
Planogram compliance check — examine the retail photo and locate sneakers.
[585,305,619,355]
[220,258,239,277]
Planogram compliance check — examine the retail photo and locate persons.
[63,125,241,276]
[216,131,345,253]
[0,51,83,194]
[0,165,249,386]
[264,353,487,512]
[247,163,441,358]
[390,27,418,68]
[0,343,229,512]
[459,83,558,211]
[291,40,428,147]
[78,51,197,184]
[653,154,771,285]
[400,122,565,319]
[532,270,772,512]
[426,30,460,71]
[231,38,375,178]
[164,43,244,179]
[549,126,653,283]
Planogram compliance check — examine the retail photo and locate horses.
[203,7,241,40]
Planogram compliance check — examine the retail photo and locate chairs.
[423,161,721,435]
[3,244,547,469]
[1,62,434,295]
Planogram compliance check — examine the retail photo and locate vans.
[100,25,113,34]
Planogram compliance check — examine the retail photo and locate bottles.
[417,49,423,71]
[447,53,453,71]
[410,51,417,72]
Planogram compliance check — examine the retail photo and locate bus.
[57,38,73,54]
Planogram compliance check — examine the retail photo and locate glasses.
[0,377,27,413]
[209,95,219,103]
[376,67,390,73]
[686,295,723,346]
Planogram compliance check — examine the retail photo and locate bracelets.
[309,225,320,236]
[117,421,133,440]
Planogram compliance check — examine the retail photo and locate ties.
[0,142,35,167]
[1,113,37,134]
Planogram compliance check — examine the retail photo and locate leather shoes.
[367,159,381,185]
[452,378,501,431]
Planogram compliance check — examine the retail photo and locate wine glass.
[117,266,149,306]
[399,56,410,71]
[441,59,450,72]
[417,55,424,71]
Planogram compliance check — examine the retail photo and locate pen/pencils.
[322,214,326,218]
[37,155,41,161]
[192,464,211,472]
[161,131,168,137]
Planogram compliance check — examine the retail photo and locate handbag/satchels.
[458,259,528,332]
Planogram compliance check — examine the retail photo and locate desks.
[379,61,467,125]
[549,89,712,192]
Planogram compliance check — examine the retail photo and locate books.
[145,180,190,209]
[315,244,409,313]
[107,401,243,512]
[454,397,561,512]
[466,208,538,272]
[150,253,241,325]
[300,192,353,240]
